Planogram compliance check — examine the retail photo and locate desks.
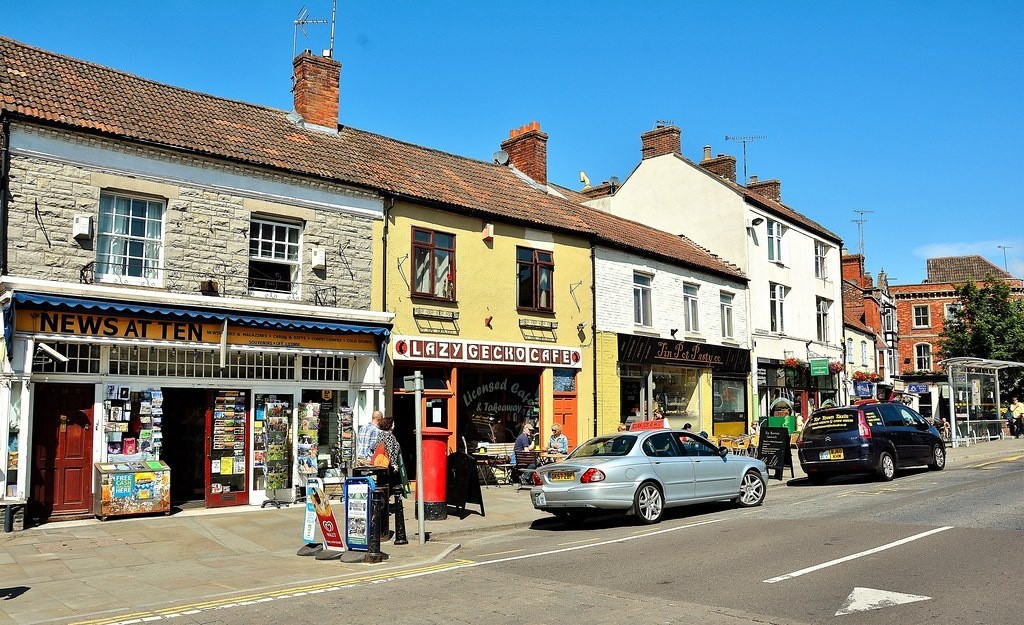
[538,453,568,464]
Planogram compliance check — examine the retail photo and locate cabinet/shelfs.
[664,403,689,415]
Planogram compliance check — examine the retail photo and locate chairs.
[468,450,552,492]
[790,434,800,450]
[707,433,760,457]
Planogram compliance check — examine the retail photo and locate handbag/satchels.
[371,436,390,468]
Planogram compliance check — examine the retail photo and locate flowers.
[851,370,883,384]
[784,358,808,376]
[827,361,846,376]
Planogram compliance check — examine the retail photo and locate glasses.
[552,429,556,432]
[528,429,532,431]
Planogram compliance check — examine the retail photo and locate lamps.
[752,218,764,226]
[311,247,326,271]
[73,215,93,240]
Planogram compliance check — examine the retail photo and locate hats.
[524,423,534,430]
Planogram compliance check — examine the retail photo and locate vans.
[797,400,947,483]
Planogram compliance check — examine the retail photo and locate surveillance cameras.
[38,343,69,363]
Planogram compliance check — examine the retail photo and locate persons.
[747,420,760,457]
[617,423,628,452]
[514,424,539,485]
[679,423,692,442]
[796,413,803,441]
[700,431,716,451]
[650,409,672,429]
[1004,397,1024,439]
[357,411,406,515]
[930,417,950,439]
[547,424,568,462]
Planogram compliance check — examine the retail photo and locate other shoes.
[520,473,530,483]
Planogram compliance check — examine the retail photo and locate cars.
[958,405,1016,437]
[529,429,769,525]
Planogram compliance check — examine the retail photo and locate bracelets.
[532,440,535,441]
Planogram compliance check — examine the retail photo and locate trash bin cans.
[353,466,392,542]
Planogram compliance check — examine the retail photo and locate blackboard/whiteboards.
[758,427,793,469]
